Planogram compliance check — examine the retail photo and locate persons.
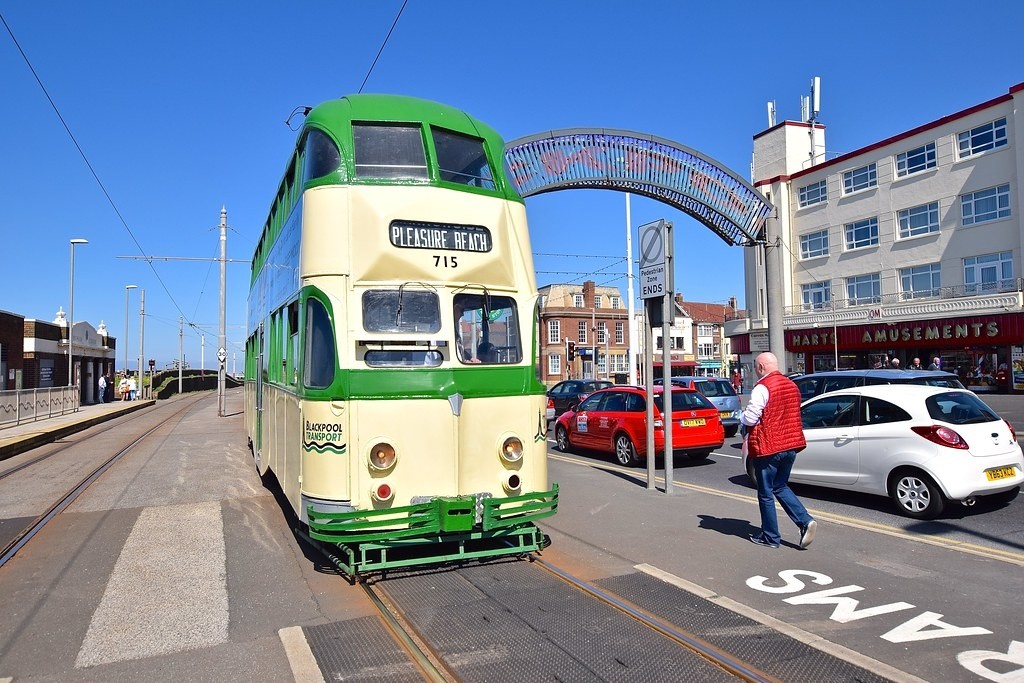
[875,353,900,369]
[425,298,483,364]
[738,352,817,549]
[118,374,136,401]
[927,357,941,386]
[732,368,742,386]
[909,357,923,370]
[98,373,113,404]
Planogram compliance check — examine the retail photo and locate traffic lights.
[567,341,577,362]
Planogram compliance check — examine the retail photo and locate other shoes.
[750,535,779,548]
[798,520,817,549]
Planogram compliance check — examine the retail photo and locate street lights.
[67,238,89,391]
[124,285,139,377]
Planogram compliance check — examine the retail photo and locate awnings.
[652,362,702,368]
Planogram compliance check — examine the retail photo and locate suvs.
[638,375,742,437]
[554,383,725,467]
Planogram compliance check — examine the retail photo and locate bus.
[238,94,562,580]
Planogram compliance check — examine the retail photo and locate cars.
[544,396,556,429]
[546,380,613,417]
[788,369,971,427]
[739,382,1024,521]
